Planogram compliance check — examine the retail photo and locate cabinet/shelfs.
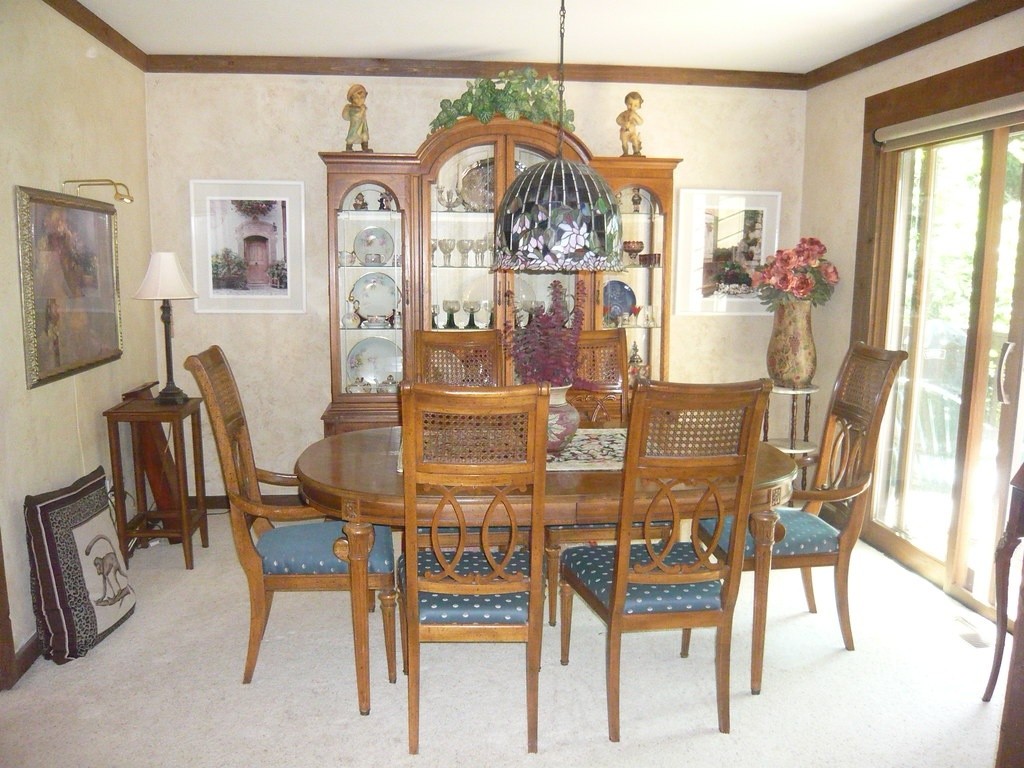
[764,383,817,508]
[319,112,684,436]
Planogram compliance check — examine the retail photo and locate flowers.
[757,237,839,311]
[503,280,601,392]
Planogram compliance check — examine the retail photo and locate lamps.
[491,0,624,273]
[134,251,198,403]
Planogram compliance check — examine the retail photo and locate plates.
[604,280,636,328]
[349,271,402,321]
[345,336,404,390]
[359,262,387,266]
[353,225,395,263]
[363,322,389,328]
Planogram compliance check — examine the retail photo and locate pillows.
[26,467,137,664]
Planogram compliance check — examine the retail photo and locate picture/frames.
[674,189,782,315]
[188,180,307,314]
[16,185,122,390]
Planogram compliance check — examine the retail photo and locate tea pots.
[374,374,401,395]
[345,377,372,393]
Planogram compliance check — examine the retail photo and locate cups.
[392,254,402,265]
[638,253,661,268]
[367,315,387,324]
[365,253,385,264]
[338,249,356,267]
[643,306,654,328]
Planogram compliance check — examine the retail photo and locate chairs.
[183,346,396,683]
[400,380,550,754]
[681,342,909,658]
[560,378,774,742]
[567,328,628,420]
[414,329,503,386]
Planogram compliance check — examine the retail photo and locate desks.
[293,420,798,717]
[103,398,210,569]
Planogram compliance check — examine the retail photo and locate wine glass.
[514,299,555,329]
[430,232,497,268]
[623,241,644,268]
[630,304,644,328]
[431,298,497,330]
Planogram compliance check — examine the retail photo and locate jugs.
[342,295,361,328]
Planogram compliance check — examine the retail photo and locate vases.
[767,296,817,389]
[543,383,582,455]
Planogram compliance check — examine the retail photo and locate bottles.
[621,312,629,327]
